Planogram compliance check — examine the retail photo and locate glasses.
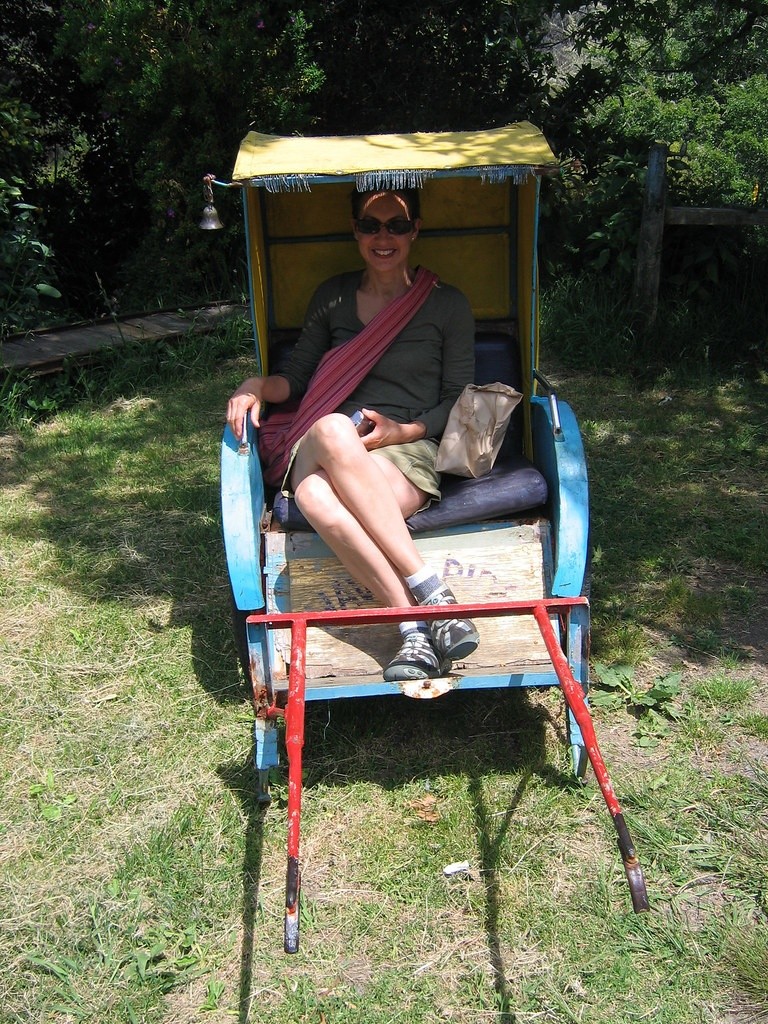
[353,215,415,235]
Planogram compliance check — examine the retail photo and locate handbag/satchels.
[435,382,524,480]
[258,409,301,485]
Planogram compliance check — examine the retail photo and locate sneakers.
[383,627,452,681]
[424,589,480,661]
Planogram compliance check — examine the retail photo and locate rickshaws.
[199,118,655,954]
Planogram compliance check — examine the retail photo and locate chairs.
[268,328,549,533]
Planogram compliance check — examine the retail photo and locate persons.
[226,181,481,680]
